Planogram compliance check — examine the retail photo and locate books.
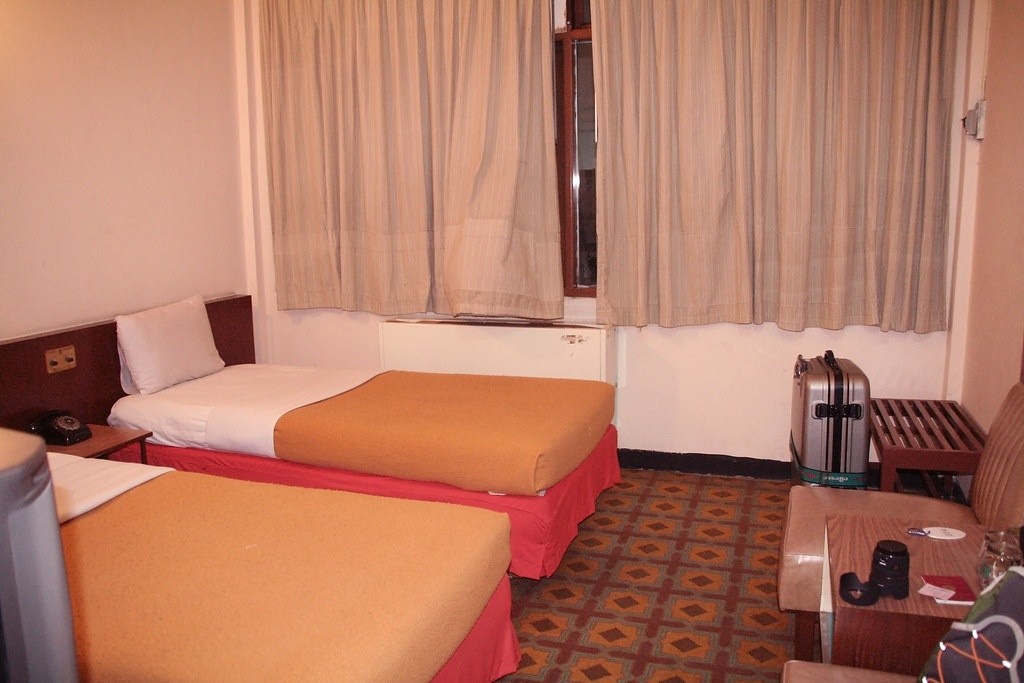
[921,575,977,605]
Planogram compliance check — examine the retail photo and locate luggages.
[788,350,873,486]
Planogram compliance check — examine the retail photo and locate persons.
[580,179,597,284]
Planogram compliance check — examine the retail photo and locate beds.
[107,363,625,581]
[46,451,523,683]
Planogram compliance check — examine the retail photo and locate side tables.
[15,424,155,466]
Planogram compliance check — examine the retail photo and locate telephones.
[25,408,93,447]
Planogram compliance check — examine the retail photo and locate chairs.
[778,381,1024,662]
[782,660,922,683]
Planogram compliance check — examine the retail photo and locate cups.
[976,528,1024,588]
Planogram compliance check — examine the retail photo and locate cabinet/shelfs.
[866,398,991,504]
[819,511,1017,677]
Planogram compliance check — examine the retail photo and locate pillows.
[115,294,228,394]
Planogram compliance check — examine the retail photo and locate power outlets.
[975,100,985,139]
